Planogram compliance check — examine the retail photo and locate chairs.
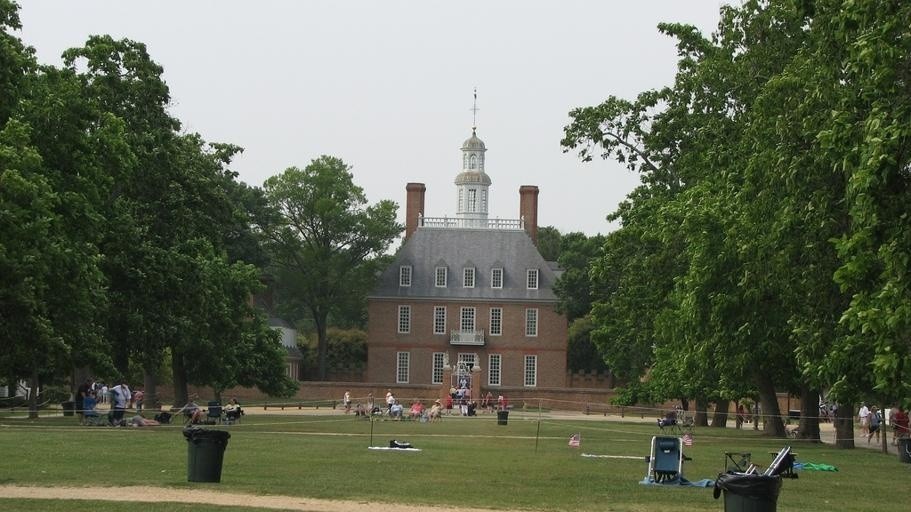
[655,411,695,438]
[180,402,241,427]
[646,434,684,486]
[356,407,440,424]
[81,397,103,427]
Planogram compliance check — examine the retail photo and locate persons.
[76,378,145,429]
[893,406,910,447]
[889,400,900,445]
[868,405,883,446]
[446,377,507,416]
[903,404,911,429]
[820,399,832,423]
[342,388,444,423]
[857,400,870,438]
[738,404,746,428]
[179,396,240,425]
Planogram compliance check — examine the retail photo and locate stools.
[723,451,799,479]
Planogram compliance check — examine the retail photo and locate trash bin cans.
[713,472,783,512]
[182,427,231,483]
[497,410,509,425]
[61,401,75,416]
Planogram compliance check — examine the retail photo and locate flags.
[681,425,695,447]
[566,432,583,449]
